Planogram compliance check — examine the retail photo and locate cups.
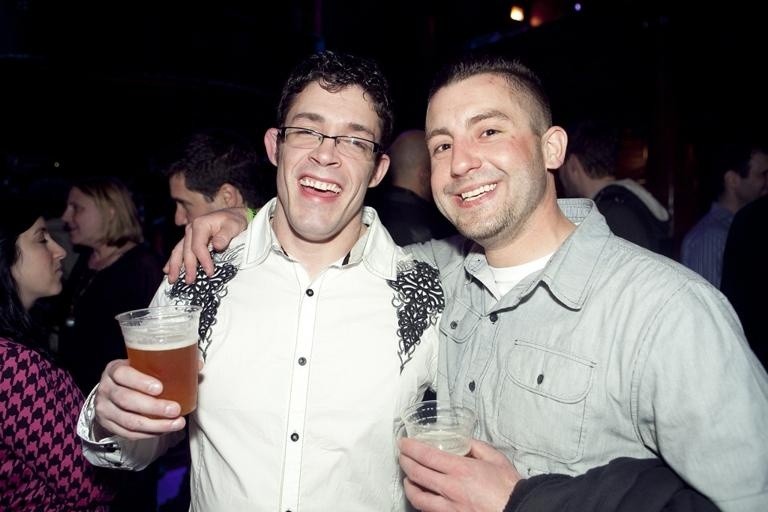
[397,400,480,460]
[115,305,204,420]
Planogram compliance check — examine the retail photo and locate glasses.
[277,124,384,160]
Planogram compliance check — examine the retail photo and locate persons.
[2,48,768,511]
